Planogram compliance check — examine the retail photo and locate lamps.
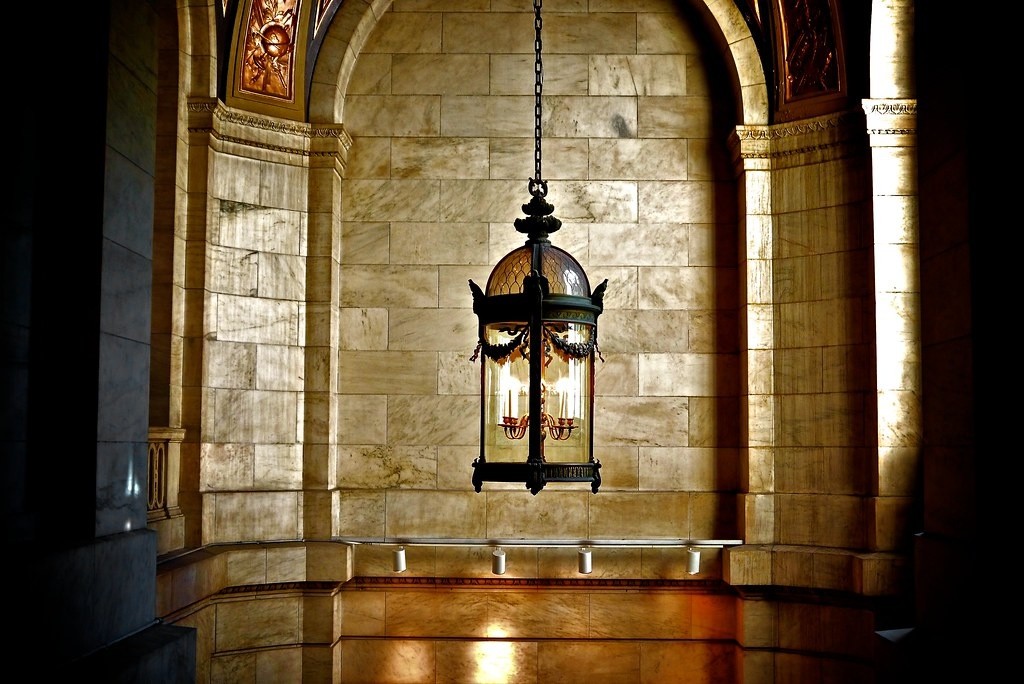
[466,0,608,494]
[392,545,407,574]
[686,547,700,574]
[492,547,507,575]
[577,547,592,573]
